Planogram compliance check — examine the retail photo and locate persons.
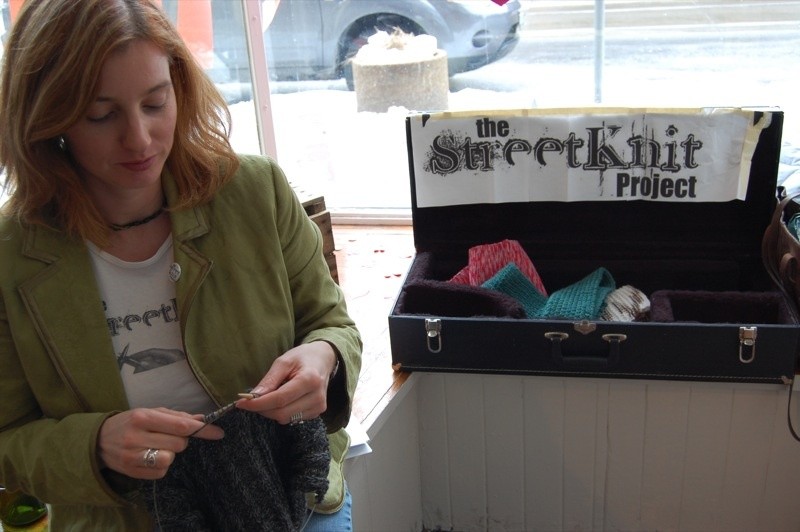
[0,0,363,532]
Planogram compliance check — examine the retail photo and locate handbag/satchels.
[772,185,800,313]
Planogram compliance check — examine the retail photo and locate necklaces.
[80,195,167,231]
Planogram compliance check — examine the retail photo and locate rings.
[290,410,304,427]
[143,448,158,467]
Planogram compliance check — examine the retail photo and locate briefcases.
[385,104,799,385]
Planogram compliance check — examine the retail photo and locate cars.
[155,0,521,92]
